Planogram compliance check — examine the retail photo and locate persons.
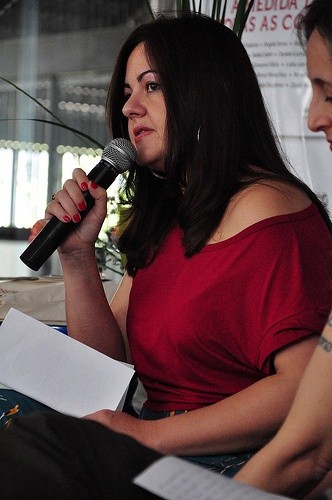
[226,0,332,496]
[31,8,332,476]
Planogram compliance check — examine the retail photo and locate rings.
[52,193,58,201]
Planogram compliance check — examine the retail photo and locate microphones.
[20,138,138,272]
